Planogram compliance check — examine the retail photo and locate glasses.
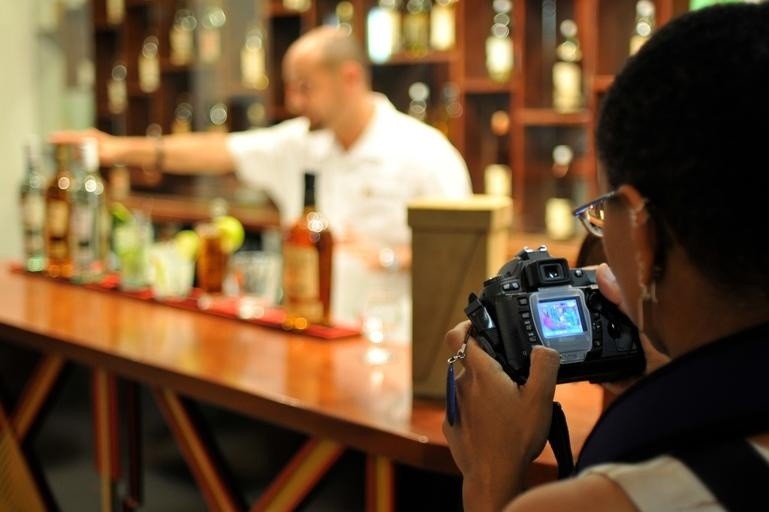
[573,190,616,238]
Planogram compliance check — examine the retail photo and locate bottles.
[15,133,110,285]
[282,169,334,326]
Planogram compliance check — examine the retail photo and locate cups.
[113,222,280,311]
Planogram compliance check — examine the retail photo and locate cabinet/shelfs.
[88,0,691,233]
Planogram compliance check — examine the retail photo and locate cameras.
[483,244,646,385]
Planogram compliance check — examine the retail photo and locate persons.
[440,3,767,510]
[43,24,476,342]
